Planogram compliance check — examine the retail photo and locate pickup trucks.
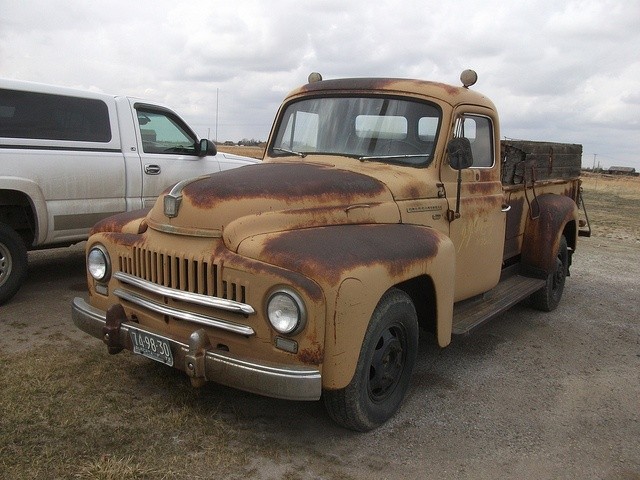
[0,78,262,307]
[71,69,591,433]
